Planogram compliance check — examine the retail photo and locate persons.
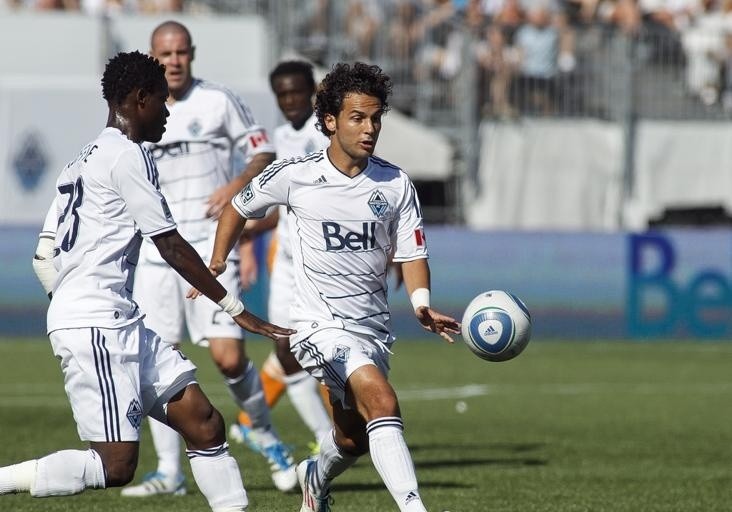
[0,50,303,512]
[229,212,336,453]
[118,22,302,500]
[227,59,336,467]
[186,62,463,512]
[267,1,731,121]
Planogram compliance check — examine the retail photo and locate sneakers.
[120,474,188,496]
[229,422,298,492]
[297,458,331,511]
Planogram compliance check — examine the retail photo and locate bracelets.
[217,291,245,318]
[410,287,432,314]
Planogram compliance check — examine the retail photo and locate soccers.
[461,290,532,361]
